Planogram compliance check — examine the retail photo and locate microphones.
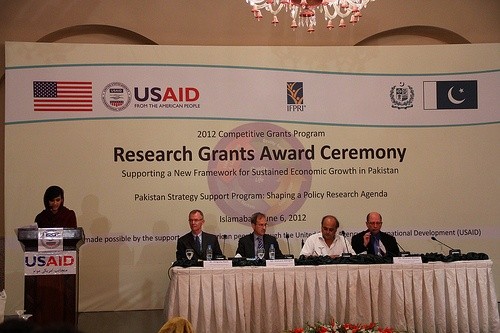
[219,234,228,260]
[286,234,294,259]
[431,236,461,254]
[381,232,409,257]
[341,231,352,257]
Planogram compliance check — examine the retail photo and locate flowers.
[282,315,405,333]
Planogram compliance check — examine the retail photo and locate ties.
[257,238,264,249]
[373,236,381,254]
[194,235,200,253]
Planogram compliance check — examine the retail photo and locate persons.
[176,210,226,266]
[299,215,357,259]
[236,213,283,257]
[352,212,401,257]
[35,186,77,228]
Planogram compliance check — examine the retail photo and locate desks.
[162,259,500,333]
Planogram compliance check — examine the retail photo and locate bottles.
[206,245,212,260]
[269,244,275,260]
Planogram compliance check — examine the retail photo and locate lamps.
[245,0,376,33]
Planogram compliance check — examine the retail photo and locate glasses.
[188,219,202,222]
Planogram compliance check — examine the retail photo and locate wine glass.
[375,246,382,256]
[186,249,194,267]
[257,248,265,259]
[318,242,325,255]
